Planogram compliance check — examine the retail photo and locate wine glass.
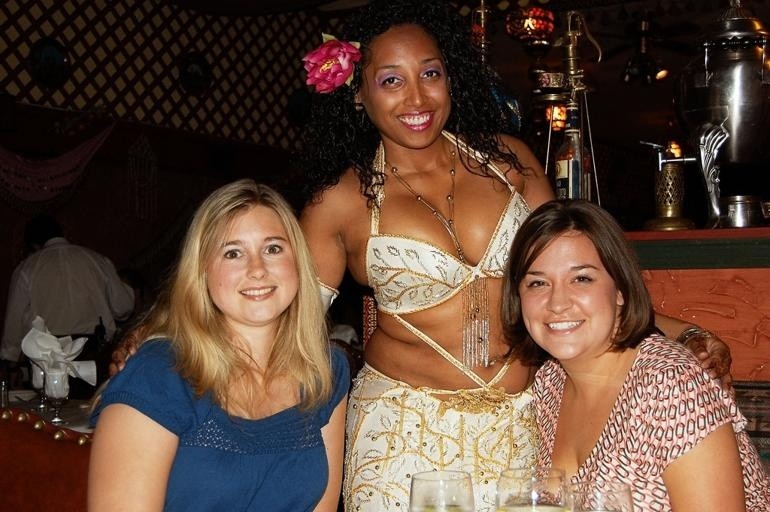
[32,357,71,424]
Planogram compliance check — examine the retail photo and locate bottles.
[727,195,757,226]
[621,20,663,108]
[554,100,591,202]
[0,379,11,408]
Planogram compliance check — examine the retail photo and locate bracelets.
[675,326,704,342]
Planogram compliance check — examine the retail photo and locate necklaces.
[376,143,469,271]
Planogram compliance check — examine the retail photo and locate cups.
[568,481,632,510]
[498,468,574,512]
[410,468,477,512]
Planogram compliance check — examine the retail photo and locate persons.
[1,215,138,396]
[494,195,770,510]
[107,0,739,510]
[80,179,353,512]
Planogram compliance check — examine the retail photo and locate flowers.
[299,34,362,92]
